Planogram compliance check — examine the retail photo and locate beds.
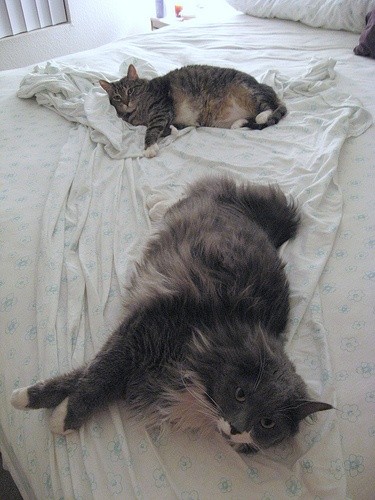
[0,0,375,499]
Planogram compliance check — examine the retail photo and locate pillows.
[227,0,375,59]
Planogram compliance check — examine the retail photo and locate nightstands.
[150,17,179,31]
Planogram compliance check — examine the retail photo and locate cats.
[10,177,334,454]
[98,63,287,157]
